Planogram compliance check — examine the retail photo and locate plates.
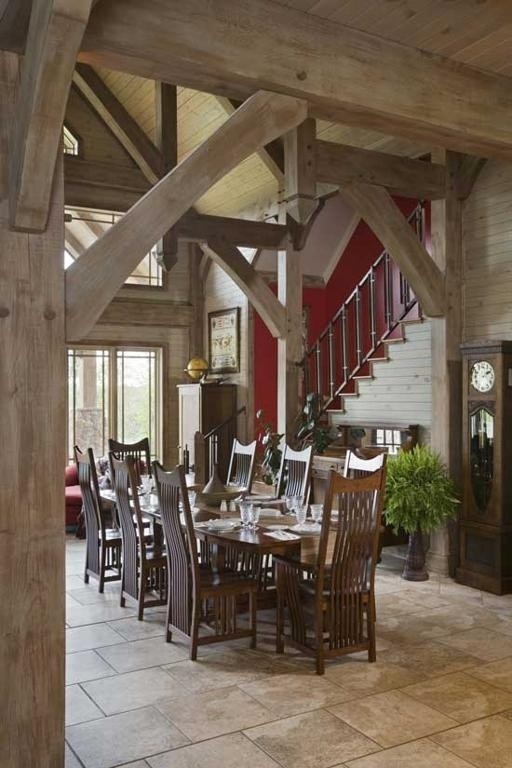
[245,495,271,502]
[204,518,242,532]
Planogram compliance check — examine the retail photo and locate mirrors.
[469,407,494,514]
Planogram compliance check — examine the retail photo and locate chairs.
[200,436,315,597]
[273,464,386,676]
[72,445,154,594]
[108,450,201,630]
[150,459,259,661]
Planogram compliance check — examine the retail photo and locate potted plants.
[378,442,462,584]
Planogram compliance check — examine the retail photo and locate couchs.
[64,461,145,526]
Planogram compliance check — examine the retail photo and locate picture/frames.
[208,306,240,374]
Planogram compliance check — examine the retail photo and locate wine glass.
[239,504,261,532]
[310,504,322,529]
[285,495,304,517]
[142,478,149,492]
[144,483,151,500]
[220,500,237,518]
[187,490,197,513]
[296,509,306,530]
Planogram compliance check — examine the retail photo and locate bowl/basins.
[186,485,248,506]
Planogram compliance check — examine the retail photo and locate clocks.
[471,361,495,393]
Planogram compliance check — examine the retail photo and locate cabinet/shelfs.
[177,384,236,483]
